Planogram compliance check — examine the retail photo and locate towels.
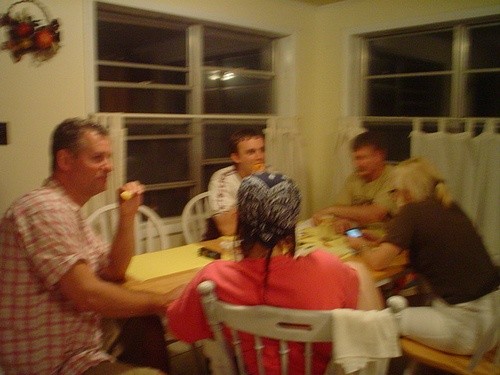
[331,307,403,375]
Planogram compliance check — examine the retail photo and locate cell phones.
[344,227,362,238]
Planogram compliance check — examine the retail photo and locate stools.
[401,338,500,375]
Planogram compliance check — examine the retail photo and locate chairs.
[197,280,407,375]
[181,191,210,245]
[85,202,169,256]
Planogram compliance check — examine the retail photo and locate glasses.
[387,188,397,196]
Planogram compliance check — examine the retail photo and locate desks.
[126,221,408,375]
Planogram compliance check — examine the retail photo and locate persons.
[201,129,279,241]
[166,171,384,375]
[311,132,396,234]
[0,117,188,375]
[341,158,500,355]
